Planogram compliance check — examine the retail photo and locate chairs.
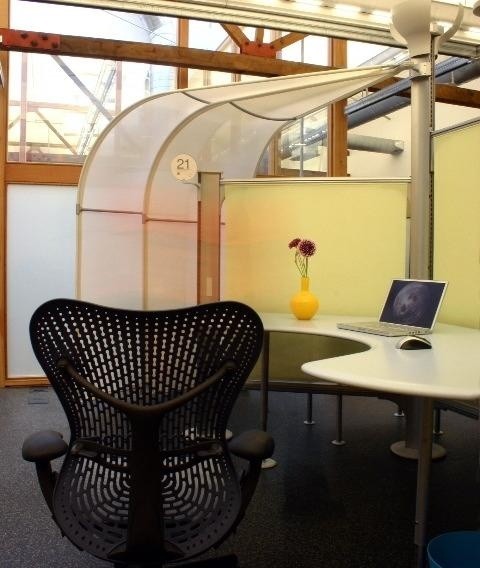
[22,299,275,566]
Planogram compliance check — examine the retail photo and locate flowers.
[288,238,313,274]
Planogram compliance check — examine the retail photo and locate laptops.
[336,279,450,338]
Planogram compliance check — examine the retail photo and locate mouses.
[395,333,432,350]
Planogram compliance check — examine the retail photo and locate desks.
[259,314,478,567]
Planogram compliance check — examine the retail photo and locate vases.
[291,272,316,319]
